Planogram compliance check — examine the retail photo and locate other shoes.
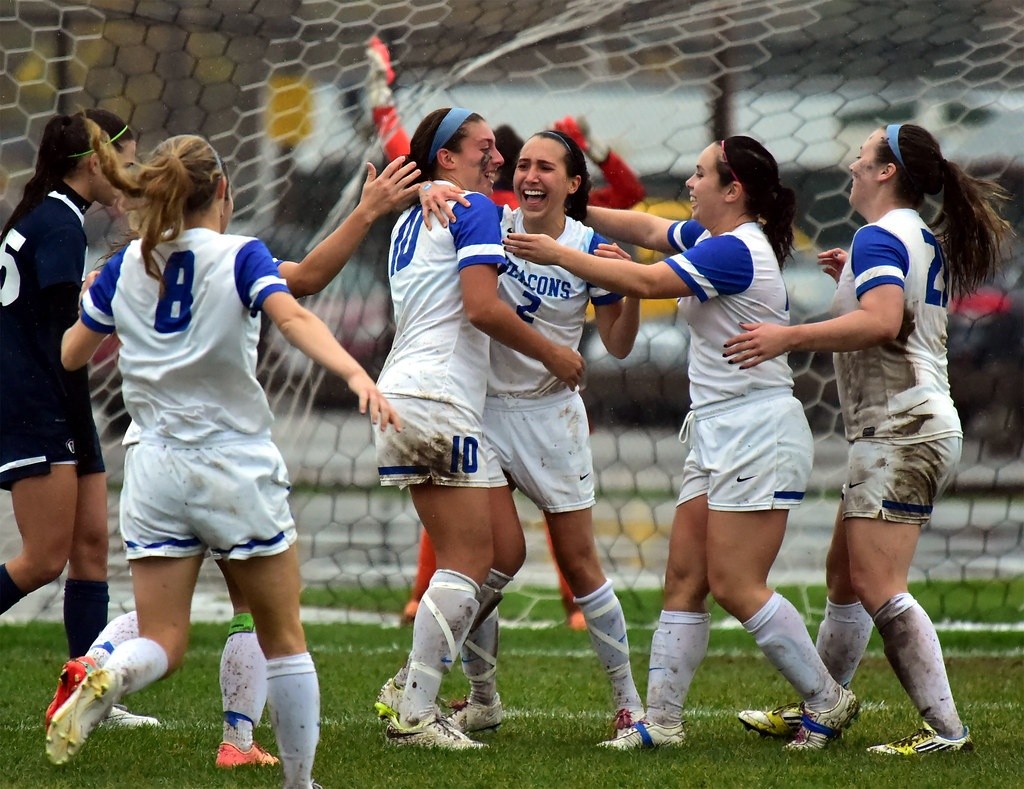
[98,705,161,731]
[311,778,323,789]
[43,668,127,766]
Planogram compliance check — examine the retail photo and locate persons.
[361,109,587,752]
[720,123,1018,755]
[44,132,401,788]
[501,131,860,762]
[46,153,425,769]
[363,37,644,631]
[407,128,644,746]
[1,109,164,735]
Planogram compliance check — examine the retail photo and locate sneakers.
[613,708,645,737]
[738,702,842,742]
[782,685,860,751]
[44,656,97,731]
[865,721,975,756]
[595,720,688,751]
[374,678,447,724]
[216,740,280,769]
[445,692,502,734]
[385,705,490,752]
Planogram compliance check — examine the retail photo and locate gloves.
[364,38,396,108]
[555,116,587,152]
